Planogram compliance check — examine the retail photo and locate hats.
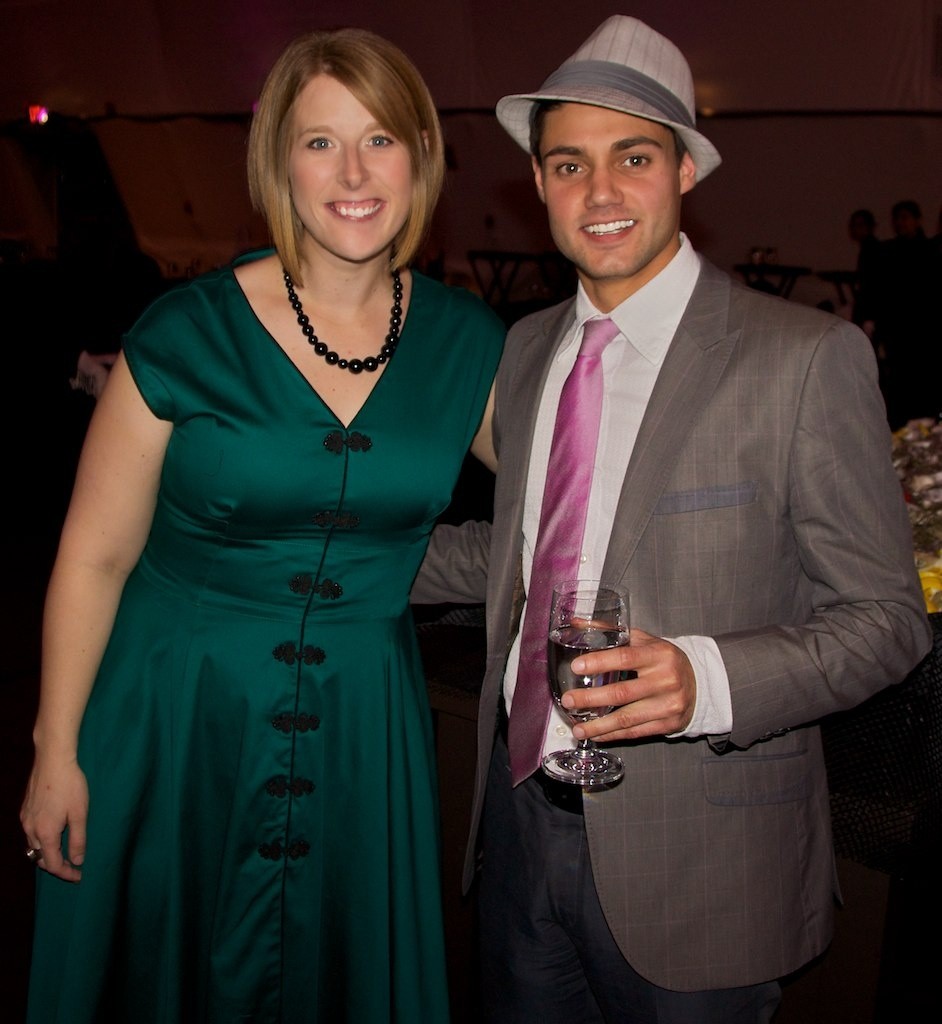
[494,13,722,191]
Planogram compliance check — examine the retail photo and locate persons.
[0,112,169,676]
[849,199,942,431]
[19,27,507,1024]
[409,15,934,1024]
[419,145,472,287]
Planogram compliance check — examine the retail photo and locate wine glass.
[542,578,630,785]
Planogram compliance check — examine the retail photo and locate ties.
[501,317,620,788]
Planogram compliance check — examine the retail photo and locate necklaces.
[283,244,403,373]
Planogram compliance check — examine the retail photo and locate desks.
[818,269,856,305]
[467,250,534,303]
[734,264,812,301]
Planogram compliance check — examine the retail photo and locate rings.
[24,848,43,863]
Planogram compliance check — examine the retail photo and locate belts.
[502,704,584,816]
